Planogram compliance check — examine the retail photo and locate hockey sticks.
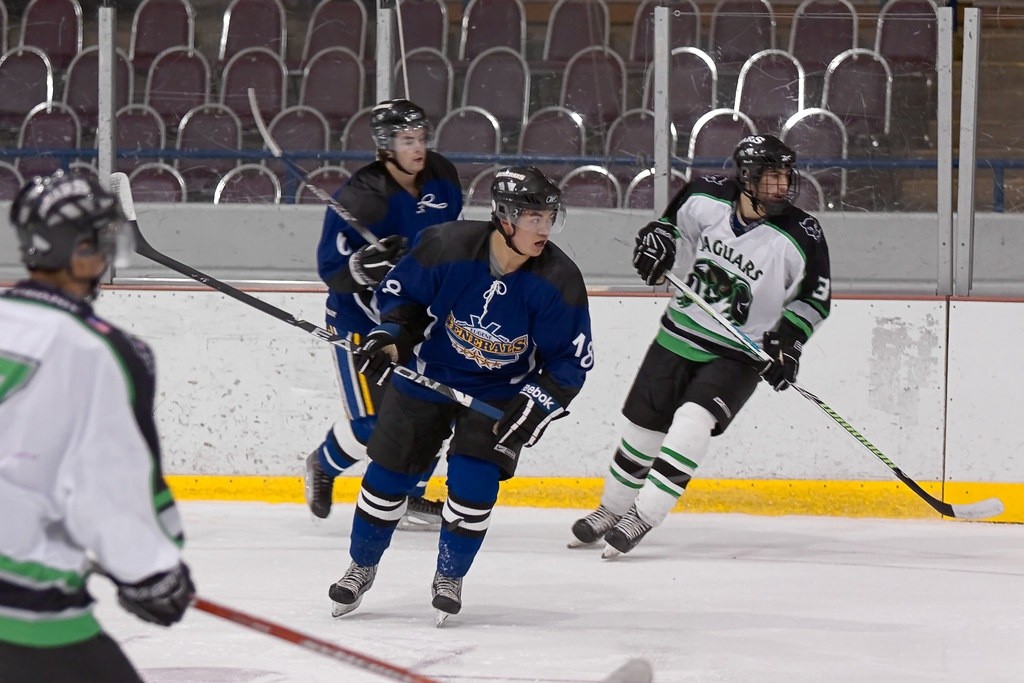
[90,558,653,683]
[663,268,1006,518]
[247,86,385,250]
[108,172,508,420]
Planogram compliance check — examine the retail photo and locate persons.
[304,99,471,530]
[0,161,196,683]
[329,166,594,629]
[566,135,831,561]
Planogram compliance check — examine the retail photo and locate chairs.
[0,0,937,212]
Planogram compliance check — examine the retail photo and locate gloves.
[119,562,196,627]
[756,332,804,392]
[352,330,400,386]
[633,220,677,286]
[493,381,570,448]
[347,235,408,288]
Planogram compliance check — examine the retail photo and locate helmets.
[734,134,800,218]
[371,98,429,152]
[490,166,562,229]
[9,168,126,274]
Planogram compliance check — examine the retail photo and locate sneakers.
[329,559,378,617]
[431,571,463,626]
[305,448,335,518]
[601,503,651,558]
[395,495,444,532]
[568,505,622,547]
